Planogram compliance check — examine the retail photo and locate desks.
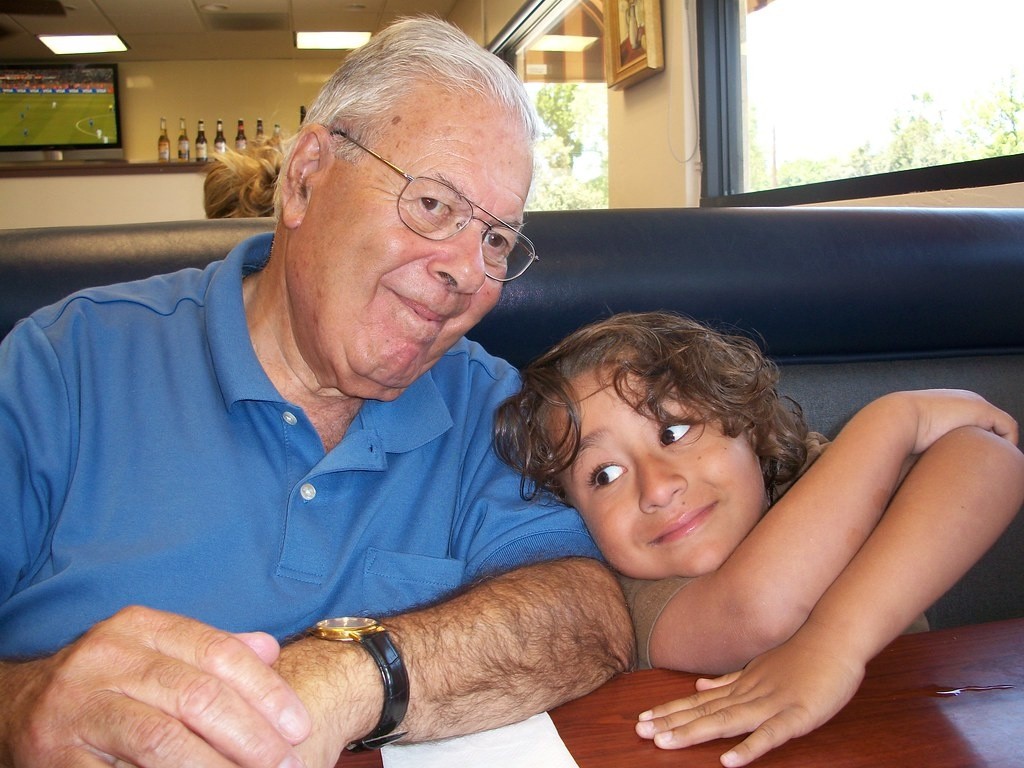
[336,615,1024,768]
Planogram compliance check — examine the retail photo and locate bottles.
[196,119,208,162]
[257,119,264,138]
[300,105,306,123]
[236,118,246,151]
[178,118,190,162]
[158,118,170,163]
[275,125,280,134]
[215,119,226,160]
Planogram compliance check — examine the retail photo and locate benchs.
[0,205,1024,631]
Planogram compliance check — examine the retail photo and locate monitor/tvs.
[0,62,122,152]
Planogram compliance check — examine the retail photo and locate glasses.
[329,129,539,282]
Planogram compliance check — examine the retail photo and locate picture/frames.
[600,0,665,93]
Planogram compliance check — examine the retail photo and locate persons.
[0,13,636,768]
[491,311,1021,768]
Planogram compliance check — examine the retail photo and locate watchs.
[305,616,411,754]
